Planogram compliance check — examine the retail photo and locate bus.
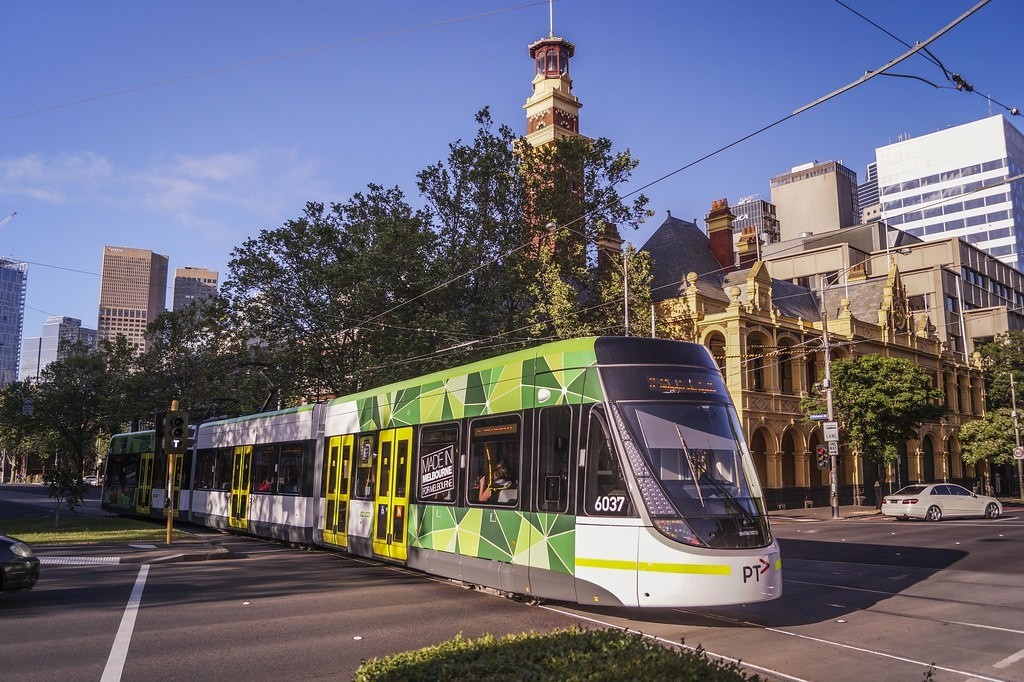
[98,337,784,611]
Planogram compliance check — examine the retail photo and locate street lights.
[818,244,914,520]
[545,224,629,336]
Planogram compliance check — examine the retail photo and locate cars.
[82,476,102,484]
[0,534,41,600]
[880,484,1003,522]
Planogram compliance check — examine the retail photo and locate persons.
[479,464,519,506]
[258,475,271,491]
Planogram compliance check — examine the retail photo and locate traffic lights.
[816,445,828,469]
[164,412,189,455]
